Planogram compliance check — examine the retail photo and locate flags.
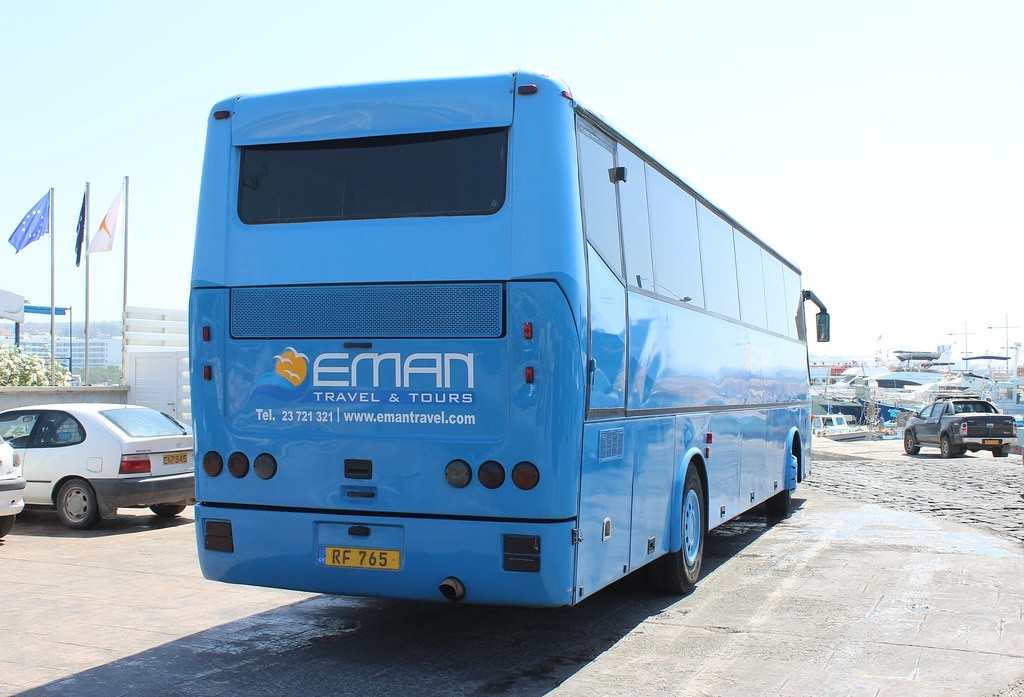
[75,193,86,267]
[87,185,124,256]
[7,192,50,254]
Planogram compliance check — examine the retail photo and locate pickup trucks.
[903,395,1018,459]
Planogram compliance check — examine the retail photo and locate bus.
[187,71,830,608]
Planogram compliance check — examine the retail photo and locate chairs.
[39,422,58,447]
[962,407,971,412]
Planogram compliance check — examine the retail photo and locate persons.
[987,397,994,409]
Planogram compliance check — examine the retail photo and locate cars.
[0,402,195,538]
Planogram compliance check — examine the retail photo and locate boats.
[810,313,1024,453]
